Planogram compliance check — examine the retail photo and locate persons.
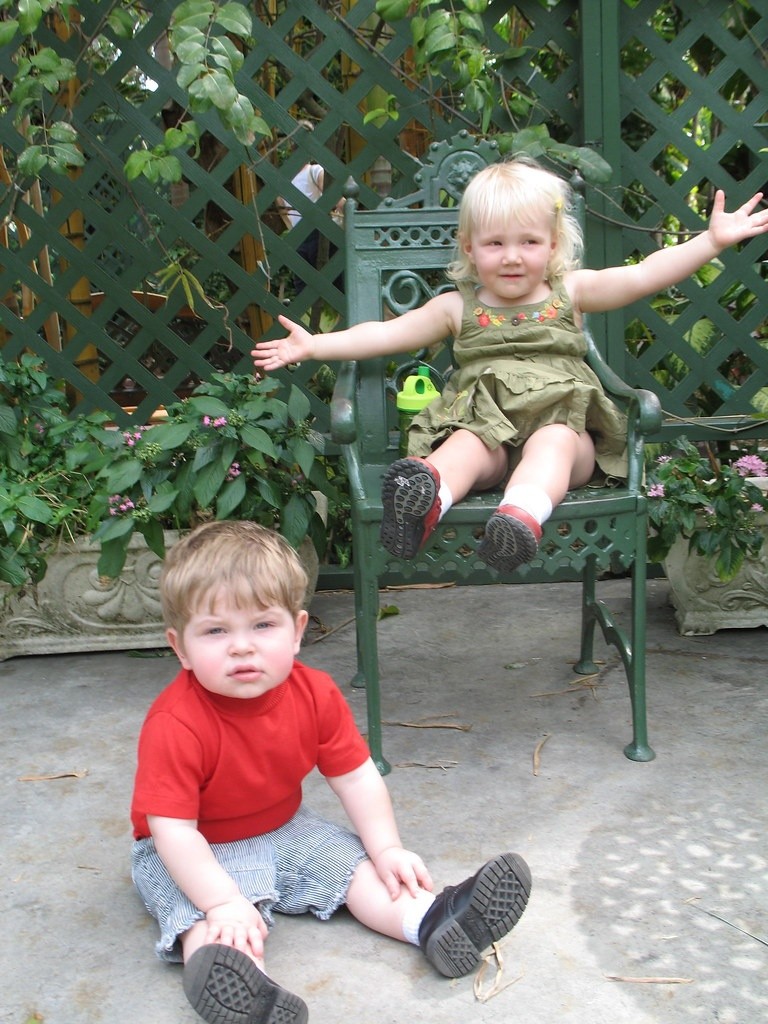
[275,120,350,298]
[130,521,532,1024]
[251,155,768,577]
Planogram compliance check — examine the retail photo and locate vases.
[0,529,317,661]
[662,477,767,636]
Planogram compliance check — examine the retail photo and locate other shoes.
[380,456,442,561]
[479,508,542,573]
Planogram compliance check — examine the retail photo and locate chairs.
[329,199,662,778]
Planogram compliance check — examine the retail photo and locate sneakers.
[182,944,310,1024]
[417,851,533,978]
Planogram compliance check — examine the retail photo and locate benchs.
[90,290,251,405]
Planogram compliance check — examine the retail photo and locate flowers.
[0,350,345,587]
[642,436,768,583]
